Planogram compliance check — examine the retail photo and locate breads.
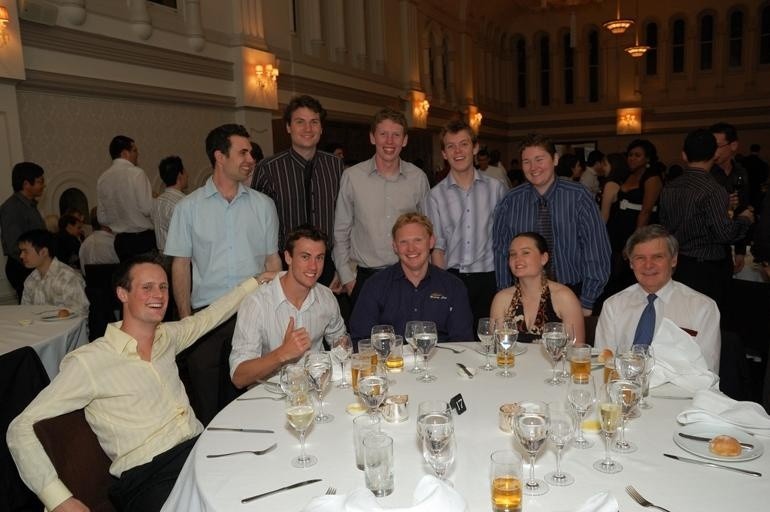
[708,434,742,457]
[58,309,69,318]
[597,348,614,364]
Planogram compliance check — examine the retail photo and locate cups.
[385,334,405,369]
[16,310,32,329]
[490,450,524,512]
[495,343,515,369]
[417,399,455,454]
[358,339,377,374]
[499,404,526,434]
[350,353,371,395]
[381,395,409,423]
[570,343,591,385]
[353,414,381,471]
[282,365,307,399]
[363,433,395,496]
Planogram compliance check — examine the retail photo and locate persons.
[1,94,769,511]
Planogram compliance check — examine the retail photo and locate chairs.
[83,260,121,314]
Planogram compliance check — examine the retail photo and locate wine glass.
[542,322,567,387]
[495,321,517,378]
[632,344,654,409]
[607,369,643,453]
[413,322,438,384]
[542,402,576,487]
[332,332,353,390]
[358,376,387,423]
[592,385,623,473]
[279,385,319,468]
[614,344,646,419]
[513,401,551,495]
[304,360,334,424]
[423,413,455,488]
[567,373,597,449]
[305,352,334,408]
[404,321,425,375]
[554,323,577,378]
[371,324,396,375]
[477,317,497,371]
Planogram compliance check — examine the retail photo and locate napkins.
[274,348,348,382]
[678,390,770,431]
[581,491,618,510]
[392,341,424,364]
[32,304,63,314]
[309,473,464,510]
[645,321,723,394]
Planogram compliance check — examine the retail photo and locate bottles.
[744,245,754,270]
[727,204,734,221]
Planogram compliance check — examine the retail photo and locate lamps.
[615,105,642,137]
[464,105,483,136]
[255,64,279,89]
[602,0,635,36]
[624,0,651,58]
[409,91,429,128]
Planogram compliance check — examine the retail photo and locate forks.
[206,444,278,458]
[237,395,288,401]
[624,486,672,512]
[435,345,466,354]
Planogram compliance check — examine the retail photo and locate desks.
[156,339,770,510]
[2,300,88,380]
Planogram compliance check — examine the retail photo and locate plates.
[672,422,764,462]
[34,310,79,322]
[265,376,285,393]
[472,342,527,357]
[566,346,606,366]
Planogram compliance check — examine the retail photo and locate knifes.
[679,432,754,451]
[241,479,322,504]
[663,454,762,477]
[456,363,473,379]
[207,427,275,434]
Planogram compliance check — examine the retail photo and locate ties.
[633,293,658,345]
[537,199,553,261]
